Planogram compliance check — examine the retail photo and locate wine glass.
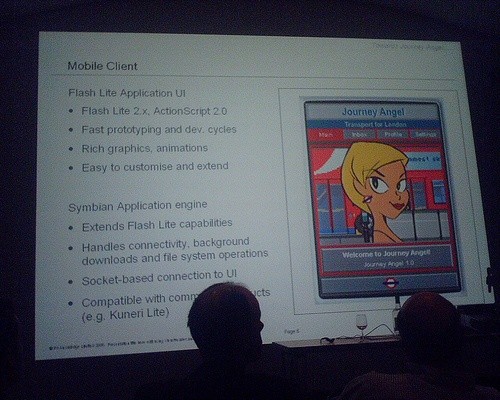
[356,314,368,341]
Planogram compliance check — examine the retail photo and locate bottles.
[392,294,402,336]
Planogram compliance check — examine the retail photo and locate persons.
[392,290,497,389]
[165,282,346,400]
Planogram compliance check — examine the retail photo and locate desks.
[272,336,405,398]
